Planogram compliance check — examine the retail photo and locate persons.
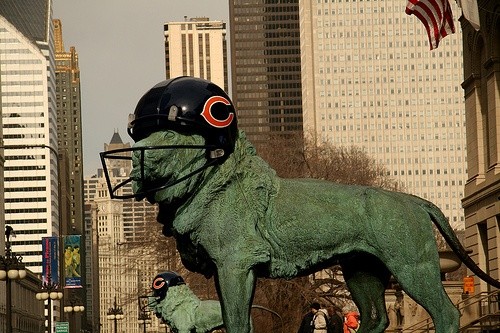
[297,302,358,333]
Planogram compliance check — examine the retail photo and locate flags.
[405,0,455,50]
[461,0,480,31]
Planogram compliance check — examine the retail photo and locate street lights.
[106,295,125,333]
[138,309,152,333]
[63,289,85,333]
[35,265,64,333]
[0,224,27,333]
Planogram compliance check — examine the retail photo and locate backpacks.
[310,311,329,333]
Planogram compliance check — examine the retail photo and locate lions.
[147,271,283,333]
[127,75,499,333]
